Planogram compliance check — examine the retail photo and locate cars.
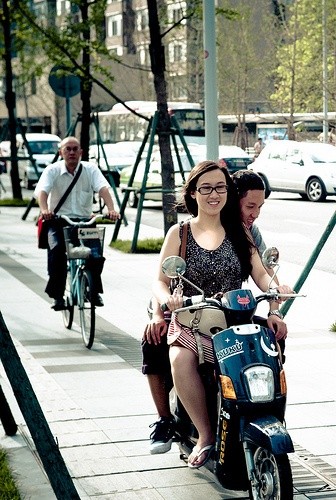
[247,140,336,202]
[119,141,253,209]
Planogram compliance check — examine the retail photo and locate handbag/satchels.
[167,308,227,364]
[36,217,49,249]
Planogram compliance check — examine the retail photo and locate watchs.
[268,310,284,320]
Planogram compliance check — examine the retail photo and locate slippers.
[188,442,214,469]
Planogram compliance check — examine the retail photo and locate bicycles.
[41,213,113,348]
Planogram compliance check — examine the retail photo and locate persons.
[151,161,296,470]
[35,137,119,310]
[253,138,266,161]
[140,169,288,454]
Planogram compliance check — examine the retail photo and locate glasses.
[61,146,80,152]
[193,184,229,195]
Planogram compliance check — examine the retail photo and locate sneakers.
[149,414,175,454]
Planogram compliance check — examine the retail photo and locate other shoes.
[55,297,65,311]
[87,291,104,306]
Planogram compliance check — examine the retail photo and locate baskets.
[63,226,106,259]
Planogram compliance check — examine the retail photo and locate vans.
[8,133,62,189]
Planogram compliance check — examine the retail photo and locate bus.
[89,100,205,188]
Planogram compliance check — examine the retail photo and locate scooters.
[147,246,294,500]
